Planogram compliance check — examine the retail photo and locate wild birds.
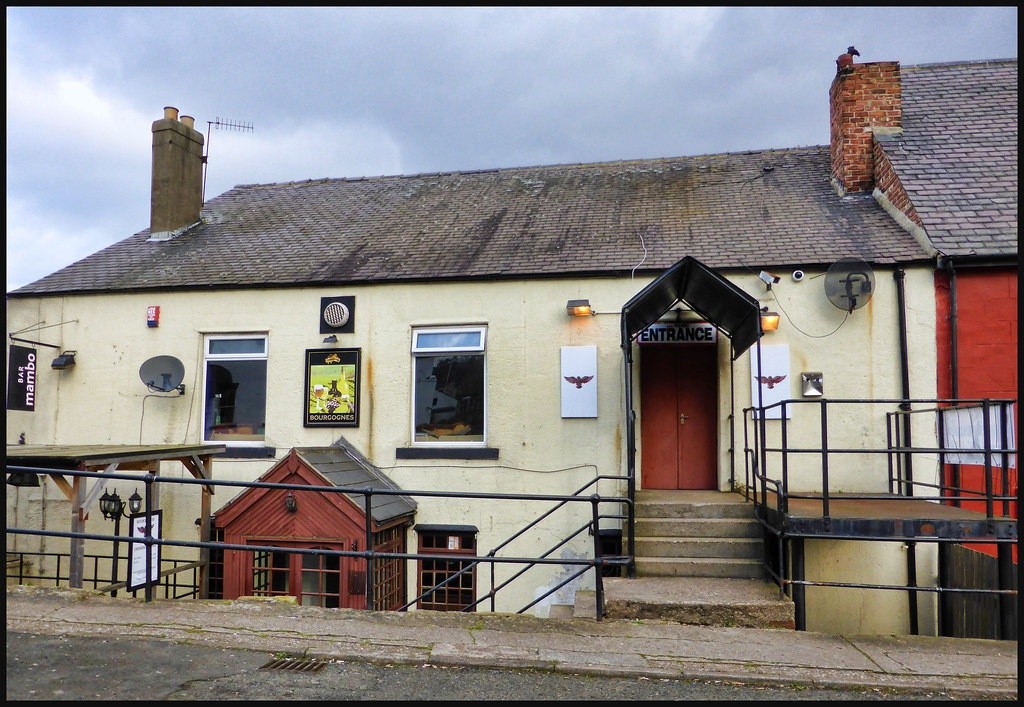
[848,46,859,56]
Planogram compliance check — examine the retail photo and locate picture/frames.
[303,347,361,428]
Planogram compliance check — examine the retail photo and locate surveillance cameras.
[793,270,804,282]
[760,271,781,284]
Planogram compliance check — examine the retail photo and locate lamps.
[566,300,591,317]
[285,492,297,512]
[6,471,41,487]
[99,486,143,521]
[50,350,77,371]
[760,312,781,331]
